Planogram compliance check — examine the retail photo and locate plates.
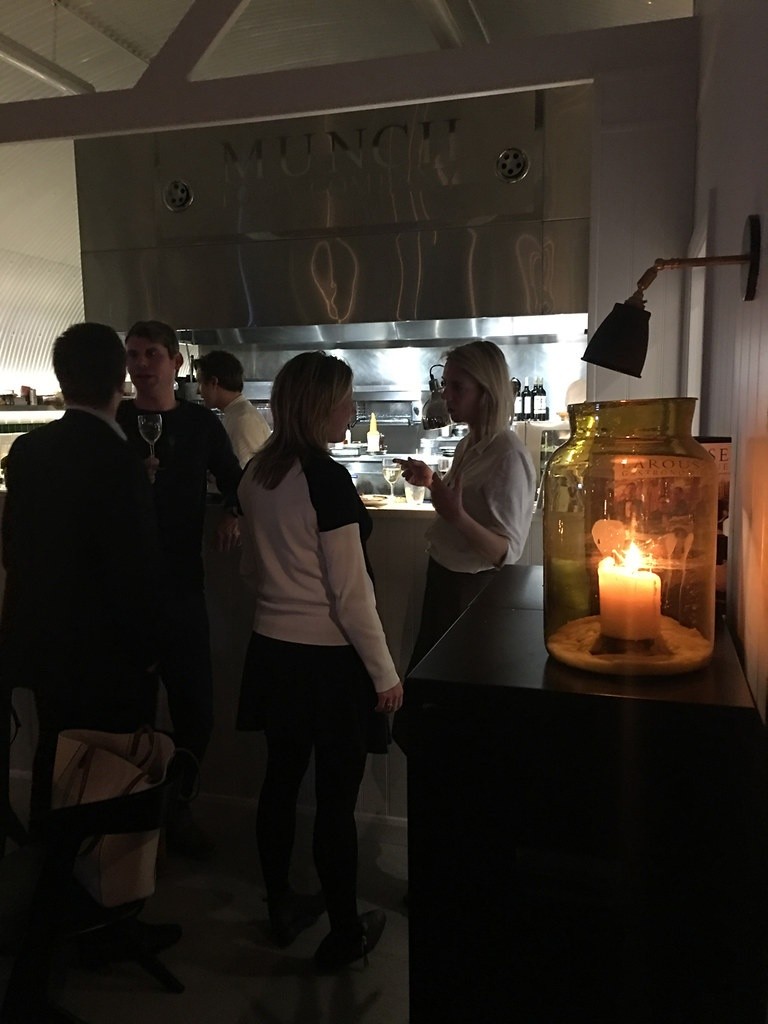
[365,450,387,455]
[360,495,388,505]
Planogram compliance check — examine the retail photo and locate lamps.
[421,364,457,430]
[581,215,763,379]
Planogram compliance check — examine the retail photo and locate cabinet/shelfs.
[193,479,414,828]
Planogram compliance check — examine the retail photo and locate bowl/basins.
[438,446,457,457]
[123,382,136,396]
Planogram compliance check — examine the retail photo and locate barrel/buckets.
[614,436,732,629]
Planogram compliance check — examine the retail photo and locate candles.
[591,519,667,638]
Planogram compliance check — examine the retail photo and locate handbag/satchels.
[51,724,175,906]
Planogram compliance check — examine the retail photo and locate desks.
[404,566,768,1024]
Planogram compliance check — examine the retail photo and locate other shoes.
[313,909,386,974]
[166,829,215,860]
[262,891,326,947]
[70,919,182,974]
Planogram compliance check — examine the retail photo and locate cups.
[437,458,452,477]
[404,480,426,506]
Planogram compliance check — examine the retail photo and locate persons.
[0,319,272,963]
[595,482,727,563]
[234,351,404,970]
[392,341,538,755]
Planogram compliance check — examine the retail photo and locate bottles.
[531,376,546,421]
[521,377,532,420]
[514,391,523,421]
[366,412,380,451]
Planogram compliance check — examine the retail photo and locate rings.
[387,703,393,706]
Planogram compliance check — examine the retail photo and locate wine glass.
[382,457,402,500]
[138,413,162,458]
